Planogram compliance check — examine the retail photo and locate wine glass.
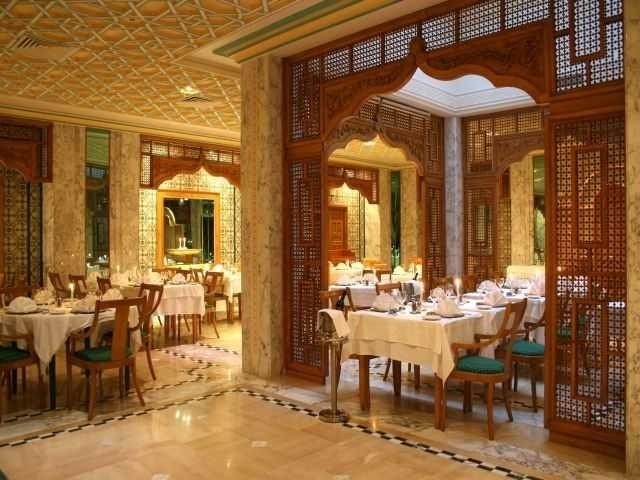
[475,278,532,292]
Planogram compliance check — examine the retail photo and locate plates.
[421,293,539,320]
[6,301,106,313]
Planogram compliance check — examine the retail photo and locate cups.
[32,287,48,305]
[168,270,176,279]
[161,272,168,281]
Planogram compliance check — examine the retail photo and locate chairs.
[1,245,224,421]
[318,257,601,412]
[440,298,528,440]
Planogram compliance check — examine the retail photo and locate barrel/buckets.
[401,281,420,304]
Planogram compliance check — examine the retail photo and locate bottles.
[411,296,421,314]
[57,296,62,307]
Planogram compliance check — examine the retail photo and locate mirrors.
[152,186,223,274]
